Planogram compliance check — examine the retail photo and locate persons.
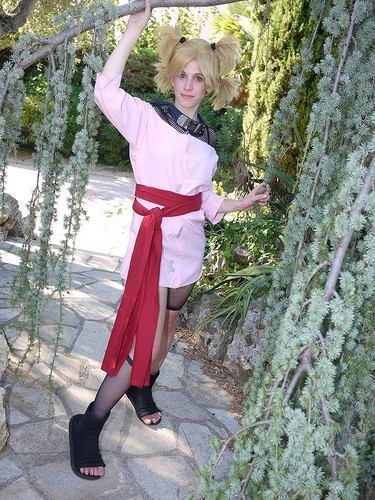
[69,0,272,481]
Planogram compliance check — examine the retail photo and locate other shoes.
[69,402,110,481]
[126,368,162,426]
